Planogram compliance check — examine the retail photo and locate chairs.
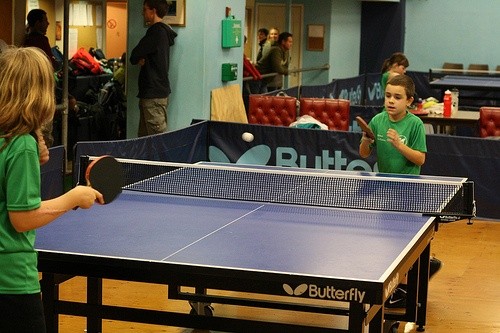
[245,95,296,129]
[495,65,500,76]
[480,107,500,139]
[468,63,488,76]
[441,63,463,75]
[300,97,350,133]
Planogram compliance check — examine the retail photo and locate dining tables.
[410,109,480,134]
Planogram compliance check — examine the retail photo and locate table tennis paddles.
[357,116,374,140]
[73,155,123,214]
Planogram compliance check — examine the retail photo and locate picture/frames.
[144,0,187,28]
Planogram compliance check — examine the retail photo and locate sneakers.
[384,283,408,309]
[429,257,441,279]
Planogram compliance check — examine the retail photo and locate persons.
[130,0,178,137]
[360,75,442,309]
[25,9,57,148]
[250,27,292,95]
[0,47,104,333]
[380,53,409,112]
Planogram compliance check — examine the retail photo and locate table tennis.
[242,132,254,142]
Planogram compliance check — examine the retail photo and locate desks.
[68,72,121,89]
[428,75,500,106]
[32,162,468,333]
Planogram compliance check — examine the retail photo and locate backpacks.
[51,46,74,75]
[97,78,124,110]
[72,48,103,76]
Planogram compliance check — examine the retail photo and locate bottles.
[417,98,422,111]
[451,87,459,116]
[113,57,118,73]
[443,89,452,117]
